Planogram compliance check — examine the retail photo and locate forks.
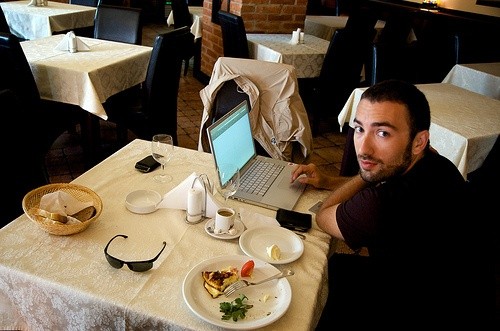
[224,267,294,298]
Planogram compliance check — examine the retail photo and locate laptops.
[206,99,308,211]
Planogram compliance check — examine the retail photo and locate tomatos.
[240,260,254,278]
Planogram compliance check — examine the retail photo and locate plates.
[239,226,305,264]
[205,216,245,240]
[182,255,292,330]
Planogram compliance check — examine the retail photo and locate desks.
[304,15,417,47]
[246,33,366,92]
[19,34,154,170]
[441,62,500,99]
[0,0,97,39]
[336,84,500,180]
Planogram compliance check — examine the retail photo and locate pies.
[202,268,239,298]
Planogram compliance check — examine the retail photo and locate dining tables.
[0,139,334,331]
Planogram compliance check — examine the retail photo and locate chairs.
[94,6,142,44]
[301,0,500,136]
[69,0,96,37]
[171,0,196,75]
[217,8,249,61]
[96,25,191,148]
[0,33,73,150]
[212,78,270,157]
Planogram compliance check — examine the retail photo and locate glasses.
[104,235,166,272]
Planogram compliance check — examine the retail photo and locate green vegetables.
[219,295,253,321]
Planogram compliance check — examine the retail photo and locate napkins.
[157,173,224,217]
[240,207,282,230]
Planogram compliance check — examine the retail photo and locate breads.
[31,209,67,224]
[68,206,96,222]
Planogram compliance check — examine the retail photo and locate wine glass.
[213,164,240,207]
[152,134,173,183]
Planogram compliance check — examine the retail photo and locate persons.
[289,80,500,331]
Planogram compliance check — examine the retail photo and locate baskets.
[22,183,103,236]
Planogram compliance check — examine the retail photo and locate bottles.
[37,0,48,7]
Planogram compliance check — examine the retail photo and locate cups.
[215,207,235,234]
